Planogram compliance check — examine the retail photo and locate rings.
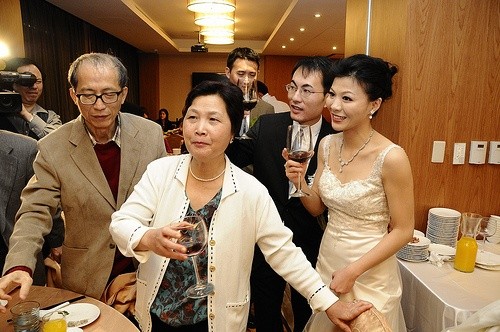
[353,298,357,304]
[283,162,287,169]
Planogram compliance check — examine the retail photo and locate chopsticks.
[6,295,86,322]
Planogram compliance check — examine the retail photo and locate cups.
[10,300,41,332]
[172,149,181,154]
[42,310,67,332]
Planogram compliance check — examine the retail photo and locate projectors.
[191,46,208,53]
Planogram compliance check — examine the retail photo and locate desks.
[398,238,500,332]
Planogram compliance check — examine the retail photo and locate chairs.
[345,298,392,332]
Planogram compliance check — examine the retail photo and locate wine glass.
[236,78,258,140]
[286,125,313,197]
[476,217,497,253]
[170,216,214,299]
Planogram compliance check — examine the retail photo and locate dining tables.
[0,284,140,332]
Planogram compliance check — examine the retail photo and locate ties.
[239,119,248,136]
[289,129,304,192]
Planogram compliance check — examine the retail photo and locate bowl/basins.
[487,215,500,244]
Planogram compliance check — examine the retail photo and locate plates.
[396,228,431,262]
[426,206,462,261]
[49,303,100,328]
[473,240,500,270]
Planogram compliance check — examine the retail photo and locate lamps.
[200,26,235,36]
[203,35,235,46]
[185,0,236,14]
[193,12,235,26]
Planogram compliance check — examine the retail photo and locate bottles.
[453,212,483,273]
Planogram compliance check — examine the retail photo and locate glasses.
[285,83,325,97]
[73,89,123,105]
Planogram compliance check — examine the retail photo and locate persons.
[0,53,169,313]
[107,80,373,332]
[0,57,64,287]
[280,54,415,332]
[132,105,186,134]
[224,48,292,177]
[180,57,342,332]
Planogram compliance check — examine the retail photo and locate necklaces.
[338,129,373,174]
[189,163,226,182]
